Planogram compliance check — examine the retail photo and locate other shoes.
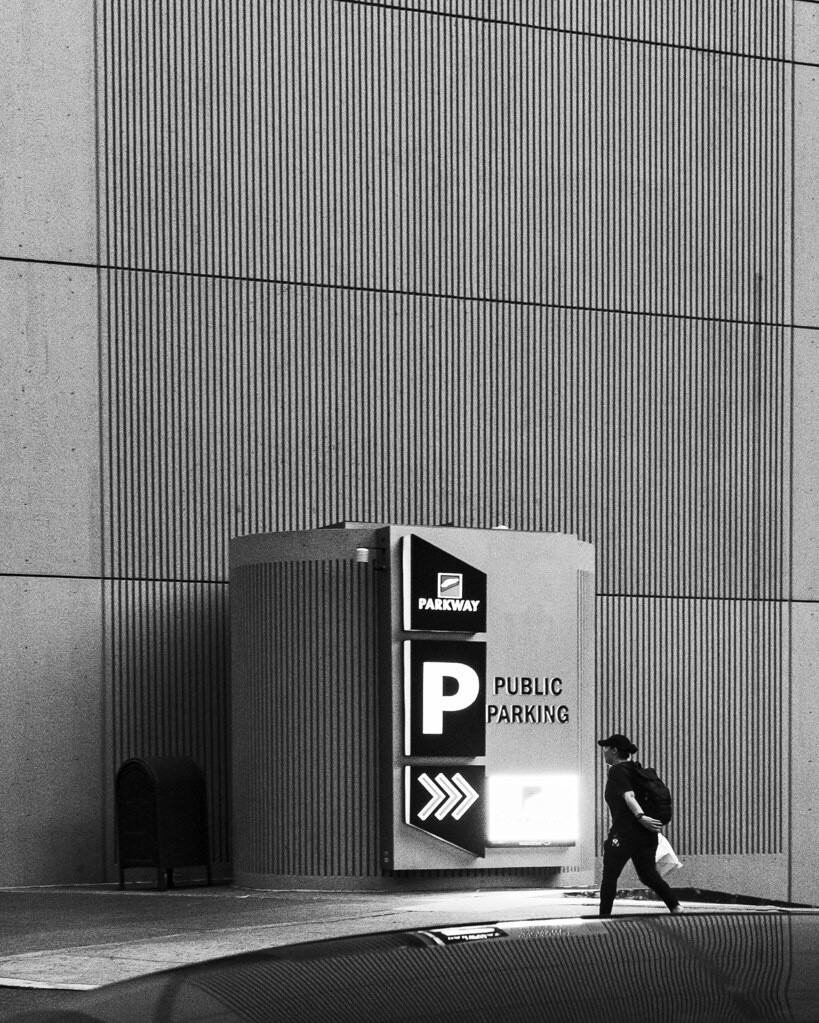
[671,905,684,915]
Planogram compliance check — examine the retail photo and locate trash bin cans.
[114,757,214,891]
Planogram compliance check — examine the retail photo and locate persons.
[598,735,685,915]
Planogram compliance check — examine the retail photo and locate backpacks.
[608,762,672,826]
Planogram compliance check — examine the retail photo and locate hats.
[598,735,634,754]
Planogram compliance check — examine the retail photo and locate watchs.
[636,813,645,822]
